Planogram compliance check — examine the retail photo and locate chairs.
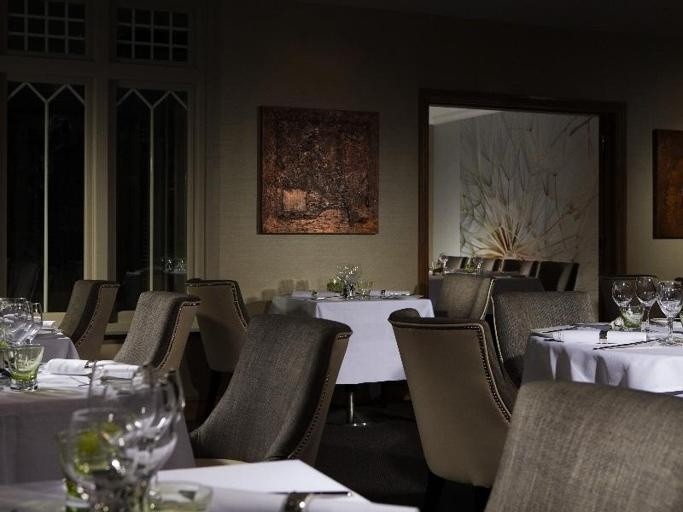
[189,313,354,469]
[387,307,513,490]
[110,288,200,368]
[436,255,585,289]
[184,275,251,376]
[482,379,683,510]
[431,272,498,320]
[490,289,597,379]
[55,277,120,362]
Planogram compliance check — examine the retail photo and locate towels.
[44,320,56,328]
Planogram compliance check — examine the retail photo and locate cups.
[57,431,119,509]
[335,263,362,299]
[70,364,212,511]
[360,281,374,297]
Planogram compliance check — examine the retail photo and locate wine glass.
[611,275,681,347]
[438,249,449,274]
[0,296,44,391]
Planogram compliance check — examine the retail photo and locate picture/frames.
[652,128,683,239]
